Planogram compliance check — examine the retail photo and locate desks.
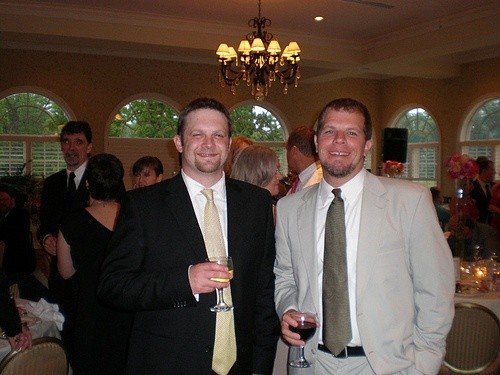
[437,278,500,375]
[0,298,73,375]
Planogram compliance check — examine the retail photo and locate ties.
[321,188,353,357]
[291,175,299,194]
[65,171,77,205]
[200,188,237,375]
[485,183,493,226]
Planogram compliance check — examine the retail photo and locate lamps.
[215,0,301,101]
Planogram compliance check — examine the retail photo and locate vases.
[449,175,475,263]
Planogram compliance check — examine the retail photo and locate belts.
[317,344,366,359]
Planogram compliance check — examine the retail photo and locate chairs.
[0,337,70,375]
[443,301,500,375]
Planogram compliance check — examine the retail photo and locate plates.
[455,288,489,297]
[21,316,42,328]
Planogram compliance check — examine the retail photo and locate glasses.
[61,140,89,147]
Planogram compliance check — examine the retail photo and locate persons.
[431,157,500,259]
[223,136,252,175]
[133,156,164,188]
[285,126,323,195]
[273,98,456,375]
[55,154,133,375]
[36,121,94,293]
[0,184,48,351]
[101,96,277,375]
[230,145,283,223]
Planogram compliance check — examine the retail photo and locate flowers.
[383,160,404,177]
[446,153,481,257]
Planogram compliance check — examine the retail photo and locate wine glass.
[207,256,234,312]
[289,312,317,367]
